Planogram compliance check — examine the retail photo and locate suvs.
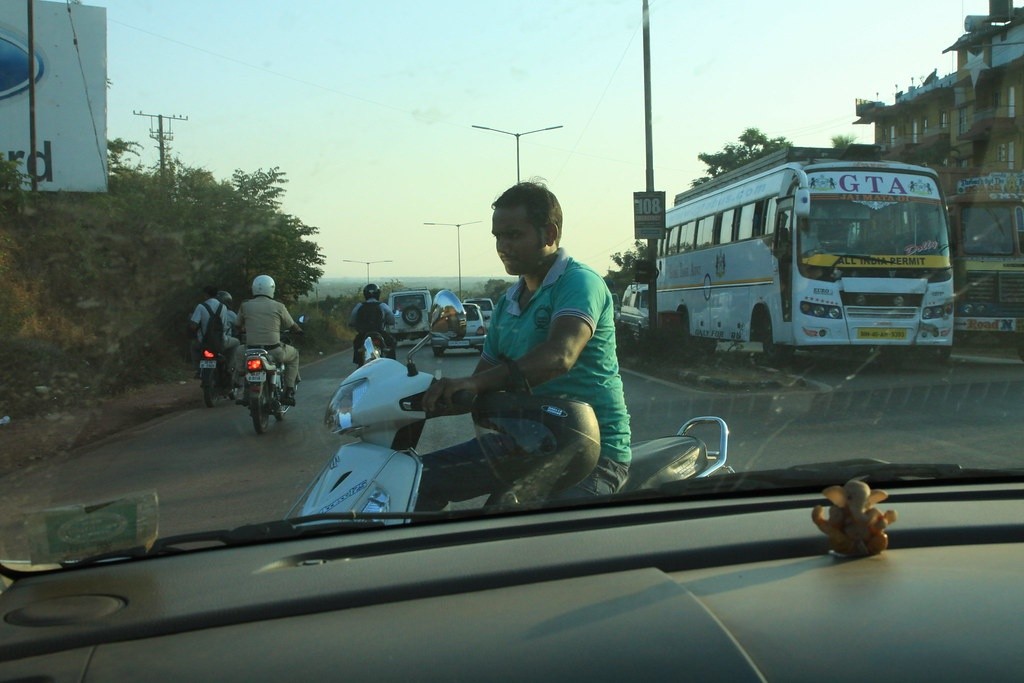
[386,290,433,344]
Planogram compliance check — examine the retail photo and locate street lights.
[424,221,486,303]
[342,259,394,285]
[470,124,563,279]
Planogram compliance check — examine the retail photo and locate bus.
[657,159,961,371]
[846,171,1023,358]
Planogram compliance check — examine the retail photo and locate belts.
[247,343,280,351]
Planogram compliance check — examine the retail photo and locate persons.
[413,182,632,511]
[802,218,823,255]
[233,275,303,406]
[349,284,396,366]
[217,291,245,387]
[188,285,241,375]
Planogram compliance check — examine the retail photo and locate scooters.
[284,289,736,526]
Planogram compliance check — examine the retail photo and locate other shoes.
[282,389,296,406]
[235,399,249,406]
[192,369,201,378]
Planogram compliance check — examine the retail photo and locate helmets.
[471,391,601,496]
[363,284,380,300]
[215,291,233,310]
[252,275,275,299]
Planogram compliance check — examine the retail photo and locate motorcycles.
[356,307,401,366]
[196,328,246,409]
[236,315,311,434]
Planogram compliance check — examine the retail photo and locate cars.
[463,297,495,329]
[609,283,649,355]
[430,303,490,357]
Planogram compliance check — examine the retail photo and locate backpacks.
[356,301,383,333]
[200,302,224,353]
